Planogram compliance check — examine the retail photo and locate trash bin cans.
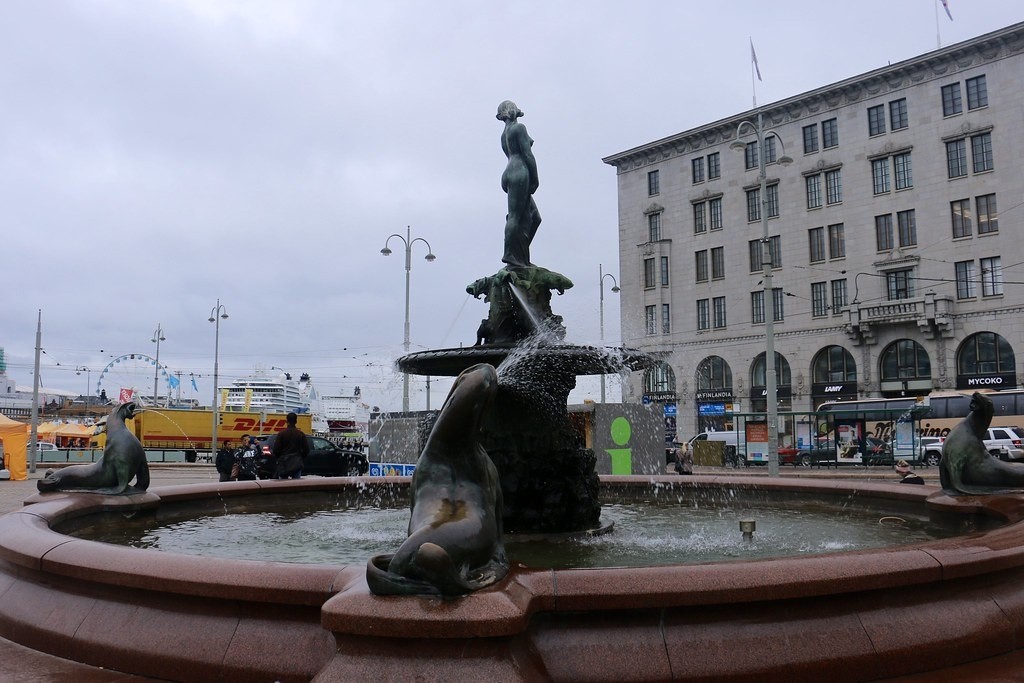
[690,440,728,468]
[723,445,737,467]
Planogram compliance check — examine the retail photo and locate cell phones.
[250,437,254,444]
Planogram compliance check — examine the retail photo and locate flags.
[751,39,762,81]
[939,0,953,21]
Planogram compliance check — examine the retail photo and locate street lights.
[207,298,229,464]
[598,263,620,404]
[150,321,166,408]
[729,112,794,479]
[380,225,437,412]
[76,366,90,429]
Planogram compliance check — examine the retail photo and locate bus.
[801,382,1024,465]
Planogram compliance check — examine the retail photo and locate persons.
[496,100,539,268]
[273,413,310,480]
[67,438,86,451]
[674,442,692,475]
[338,440,363,453]
[840,430,858,457]
[215,434,272,482]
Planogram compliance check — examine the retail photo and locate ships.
[217,368,330,433]
[320,385,370,441]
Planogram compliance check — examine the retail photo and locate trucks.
[685,429,746,467]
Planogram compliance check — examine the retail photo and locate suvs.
[250,433,370,479]
[982,425,1024,462]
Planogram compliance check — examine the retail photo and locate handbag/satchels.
[279,452,304,476]
[674,460,684,472]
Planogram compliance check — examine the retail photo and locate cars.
[876,435,946,467]
[797,436,894,467]
[26,442,59,452]
[666,441,685,465]
[777,436,833,466]
